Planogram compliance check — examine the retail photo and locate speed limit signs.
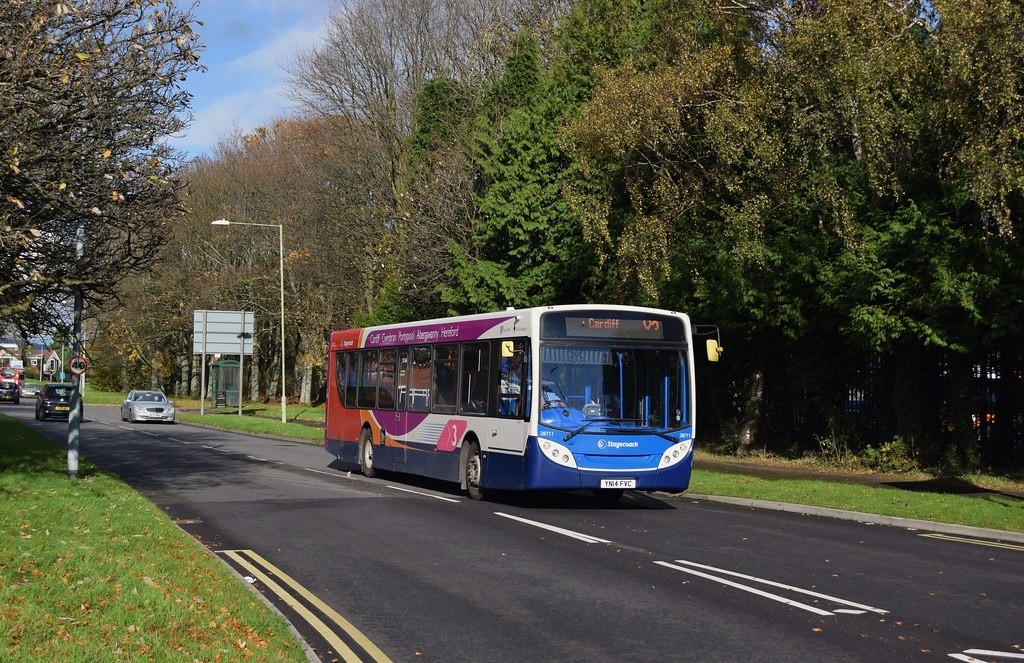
[69,357,88,374]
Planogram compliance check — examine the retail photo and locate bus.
[323,305,724,500]
[0,367,20,387]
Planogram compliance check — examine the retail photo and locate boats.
[212,219,287,423]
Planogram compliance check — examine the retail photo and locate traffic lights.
[49,359,55,371]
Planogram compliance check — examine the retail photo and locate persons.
[499,355,565,415]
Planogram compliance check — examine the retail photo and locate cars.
[0,382,19,404]
[122,389,176,423]
[34,384,84,422]
[19,383,40,398]
